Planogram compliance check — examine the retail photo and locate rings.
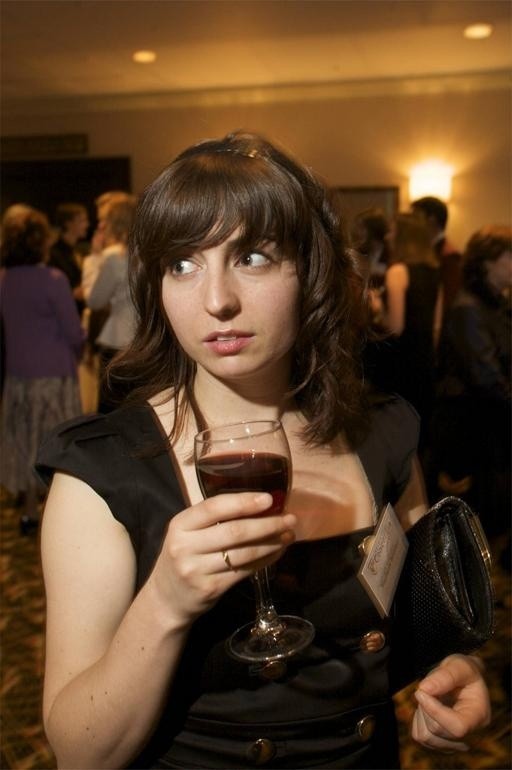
[220,550,232,570]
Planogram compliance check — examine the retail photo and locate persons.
[352,196,510,540]
[32,131,509,767]
[2,191,141,541]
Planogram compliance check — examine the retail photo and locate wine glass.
[192,418,317,665]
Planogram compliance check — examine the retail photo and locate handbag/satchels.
[382,497,495,692]
